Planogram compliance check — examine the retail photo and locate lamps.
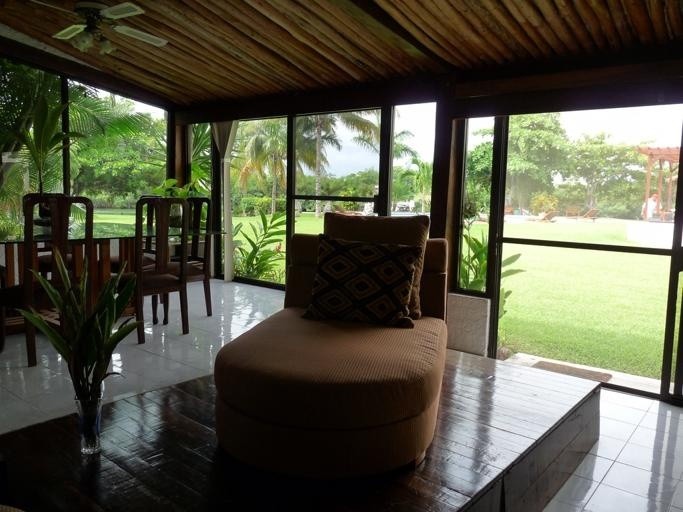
[71,20,117,54]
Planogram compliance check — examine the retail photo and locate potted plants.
[15,241,145,455]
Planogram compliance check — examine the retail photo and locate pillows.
[300,212,431,330]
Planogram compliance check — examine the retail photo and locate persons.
[639,193,664,220]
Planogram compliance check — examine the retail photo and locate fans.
[27,1,167,54]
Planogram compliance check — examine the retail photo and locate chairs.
[1,191,212,368]
[212,233,447,477]
